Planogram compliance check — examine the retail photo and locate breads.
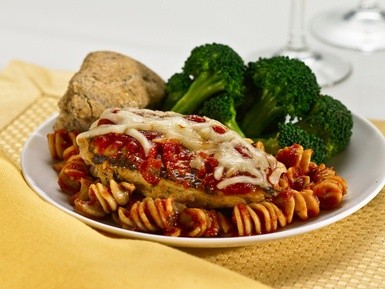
[77,106,288,208]
[54,51,167,132]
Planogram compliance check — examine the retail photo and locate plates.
[20,110,385,249]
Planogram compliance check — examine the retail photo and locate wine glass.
[309,0,385,54]
[272,0,353,89]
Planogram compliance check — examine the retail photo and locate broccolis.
[246,55,353,166]
[164,43,246,138]
